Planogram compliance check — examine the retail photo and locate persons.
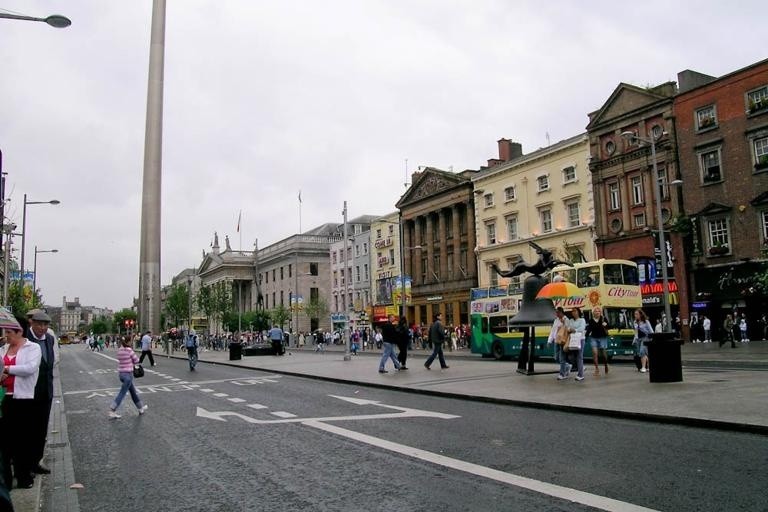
[655,310,768,348]
[349,314,469,373]
[586,307,609,377]
[199,324,346,357]
[85,326,199,372]
[96,335,148,418]
[0,306,59,512]
[630,308,654,373]
[547,306,573,379]
[569,306,586,380]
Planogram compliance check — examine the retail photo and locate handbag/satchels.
[563,335,569,352]
[133,366,144,377]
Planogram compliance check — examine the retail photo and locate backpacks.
[186,335,196,348]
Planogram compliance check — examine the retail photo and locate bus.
[176,316,207,333]
[465,257,645,362]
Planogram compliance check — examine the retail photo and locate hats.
[26,309,50,322]
[555,307,564,313]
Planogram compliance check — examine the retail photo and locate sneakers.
[557,367,586,380]
[109,412,122,418]
[138,406,148,414]
[424,364,431,369]
[395,364,408,370]
[441,366,448,368]
[379,370,388,372]
[639,368,645,373]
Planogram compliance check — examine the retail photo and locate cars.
[57,332,87,345]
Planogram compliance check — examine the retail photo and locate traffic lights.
[130,319,133,328]
[125,319,129,329]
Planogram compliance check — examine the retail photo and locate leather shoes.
[33,464,52,475]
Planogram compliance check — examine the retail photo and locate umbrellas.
[535,280,585,318]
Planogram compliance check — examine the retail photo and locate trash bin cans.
[167,339,173,355]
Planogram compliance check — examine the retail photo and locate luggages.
[634,343,647,370]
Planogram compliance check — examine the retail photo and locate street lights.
[236,276,243,333]
[183,274,195,332]
[279,251,311,349]
[377,210,422,320]
[16,195,62,306]
[614,123,684,336]
[30,245,58,307]
[1,9,73,28]
